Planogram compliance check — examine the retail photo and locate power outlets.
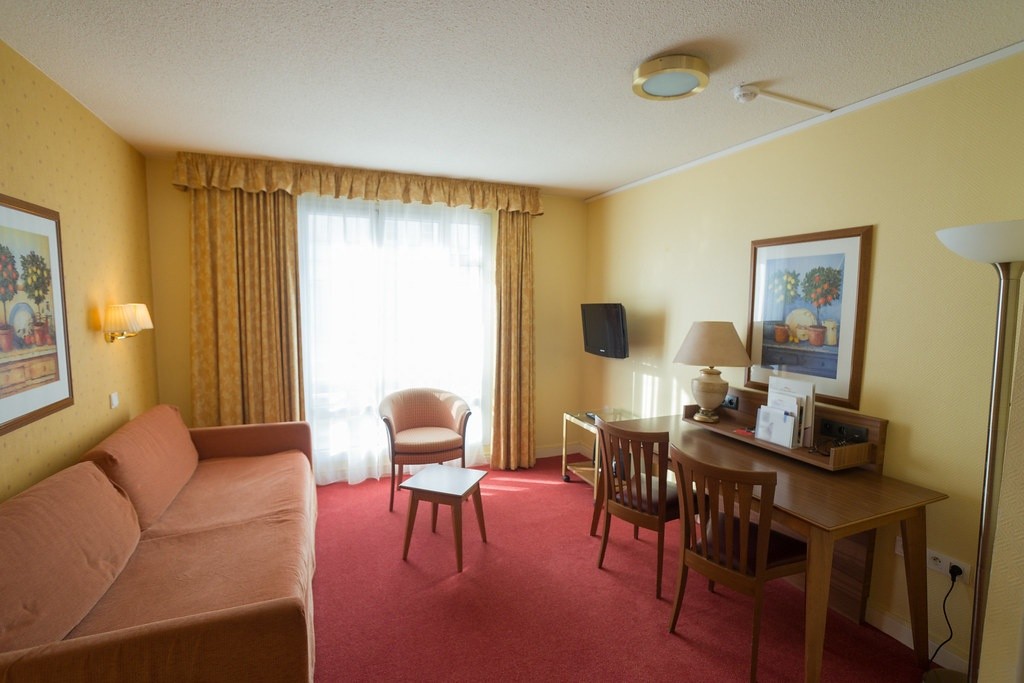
[821,418,868,444]
[894,536,974,586]
[721,395,738,410]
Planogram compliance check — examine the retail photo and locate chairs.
[594,415,709,599]
[669,444,806,683]
[379,388,472,512]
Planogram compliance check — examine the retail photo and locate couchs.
[0,403,319,683]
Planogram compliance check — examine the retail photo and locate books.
[753,375,816,449]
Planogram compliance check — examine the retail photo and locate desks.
[590,413,949,683]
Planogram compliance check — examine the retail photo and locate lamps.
[632,54,709,102]
[673,321,754,424]
[103,304,154,345]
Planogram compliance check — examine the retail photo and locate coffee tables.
[398,464,488,573]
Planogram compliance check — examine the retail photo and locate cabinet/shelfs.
[561,408,642,495]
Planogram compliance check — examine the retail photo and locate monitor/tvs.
[581,302,629,358]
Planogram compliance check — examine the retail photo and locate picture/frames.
[0,193,75,437]
[744,225,874,411]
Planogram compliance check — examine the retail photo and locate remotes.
[586,412,596,420]
